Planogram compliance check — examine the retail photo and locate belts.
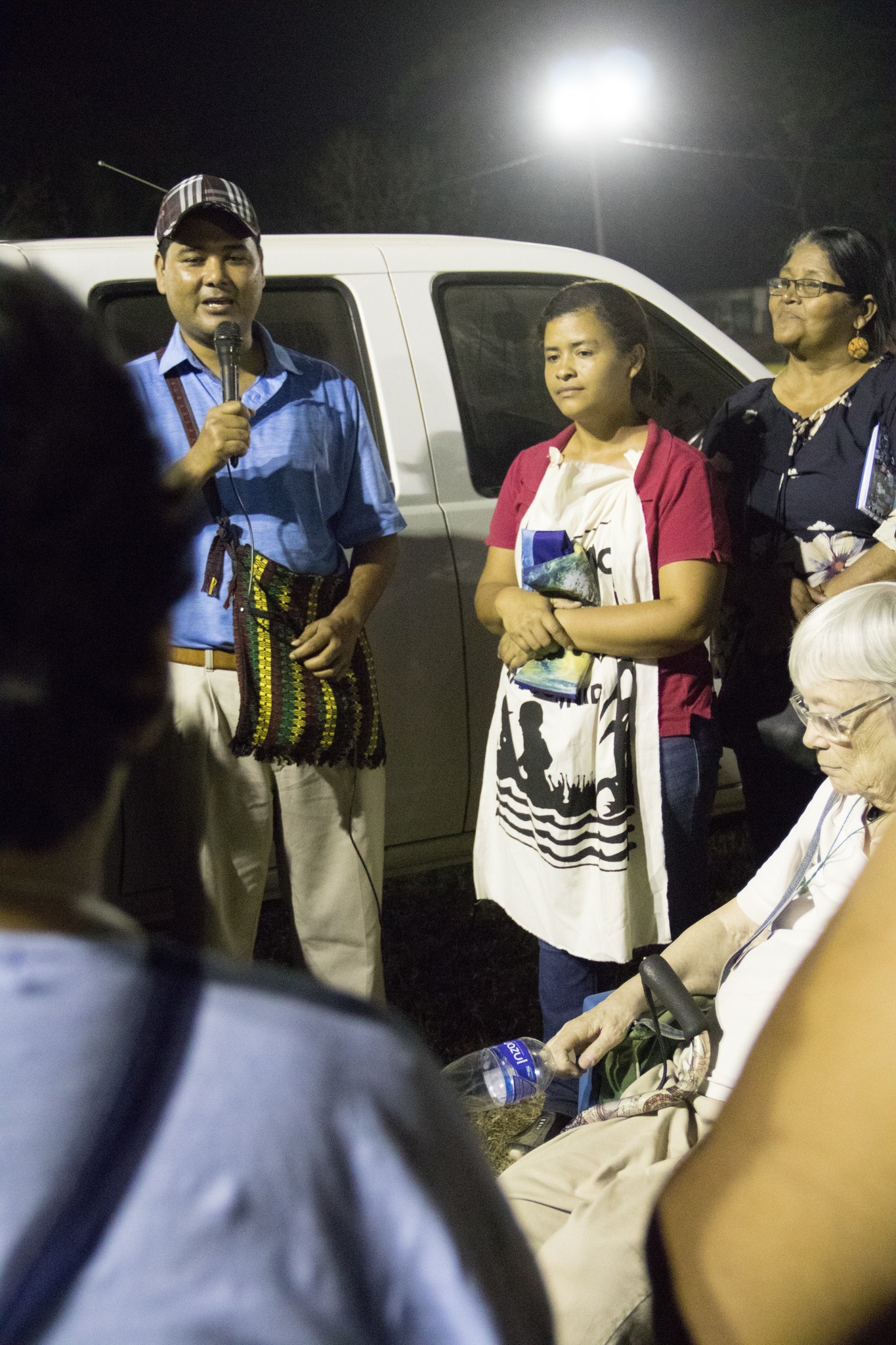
[166,645,238,670]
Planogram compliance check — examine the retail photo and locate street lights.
[549,53,646,250]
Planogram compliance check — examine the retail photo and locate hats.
[154,174,260,245]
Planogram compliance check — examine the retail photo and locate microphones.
[213,320,242,468]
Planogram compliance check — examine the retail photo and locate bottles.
[439,1036,576,1115]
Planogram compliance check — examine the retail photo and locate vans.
[0,229,781,922]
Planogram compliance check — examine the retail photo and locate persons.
[129,174,408,1004]
[495,581,896,1345]
[472,281,730,1159]
[645,818,896,1345]
[0,262,558,1345]
[686,226,896,867]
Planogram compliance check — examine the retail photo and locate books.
[855,424,896,524]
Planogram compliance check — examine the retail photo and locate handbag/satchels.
[152,345,388,771]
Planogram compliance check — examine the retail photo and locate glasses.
[788,693,892,741]
[767,275,852,299]
